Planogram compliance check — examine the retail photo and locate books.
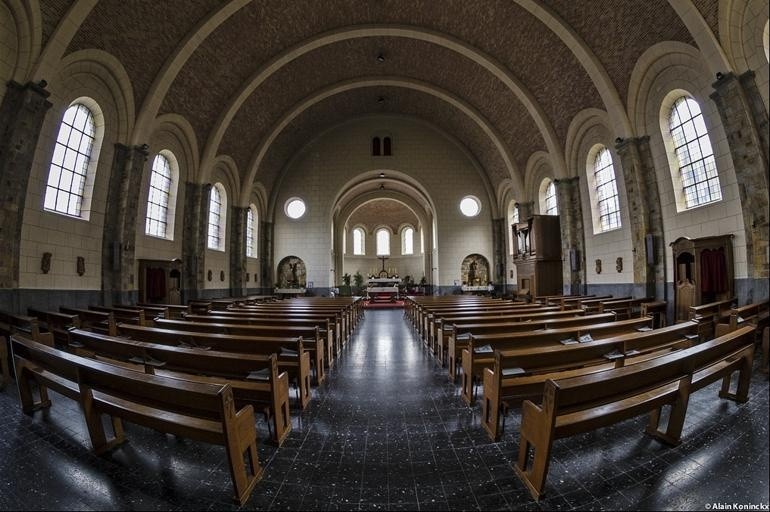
[501,367,525,376]
[685,334,699,339]
[637,325,653,332]
[625,349,640,357]
[559,332,594,345]
[279,346,298,357]
[456,332,473,340]
[603,349,624,360]
[68,341,84,348]
[177,340,212,352]
[246,367,270,380]
[474,344,494,353]
[128,351,166,367]
